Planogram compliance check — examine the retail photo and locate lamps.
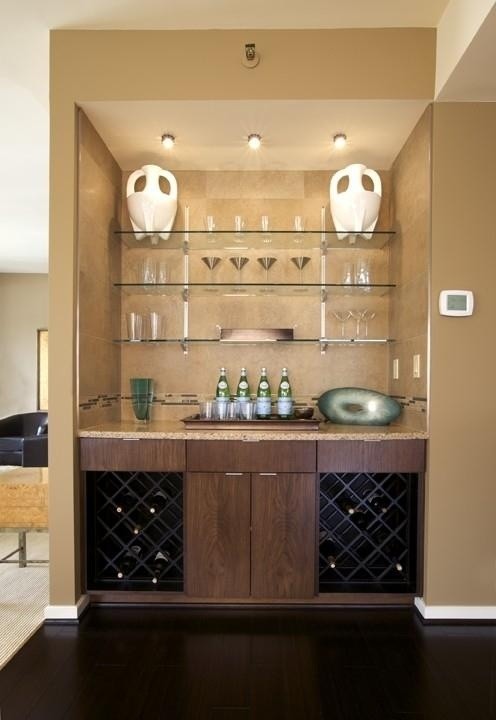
[333,134,347,149]
[247,134,263,149]
[160,134,176,149]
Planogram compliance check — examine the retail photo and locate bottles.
[236,368,249,401]
[342,255,375,295]
[277,368,293,419]
[256,367,272,419]
[138,255,172,290]
[216,367,230,401]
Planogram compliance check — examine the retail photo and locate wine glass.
[229,256,249,292]
[201,255,223,291]
[257,257,276,292]
[291,255,311,291]
[331,307,376,343]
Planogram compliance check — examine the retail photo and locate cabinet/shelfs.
[182,441,315,600]
[80,438,183,593]
[110,206,398,355]
[315,441,428,607]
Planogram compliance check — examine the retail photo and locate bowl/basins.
[293,406,315,418]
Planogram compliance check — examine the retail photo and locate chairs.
[0,410,48,466]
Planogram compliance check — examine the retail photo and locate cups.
[203,215,219,243]
[125,312,144,342]
[232,216,248,242]
[258,215,272,242]
[199,401,254,420]
[147,311,165,341]
[292,214,307,240]
